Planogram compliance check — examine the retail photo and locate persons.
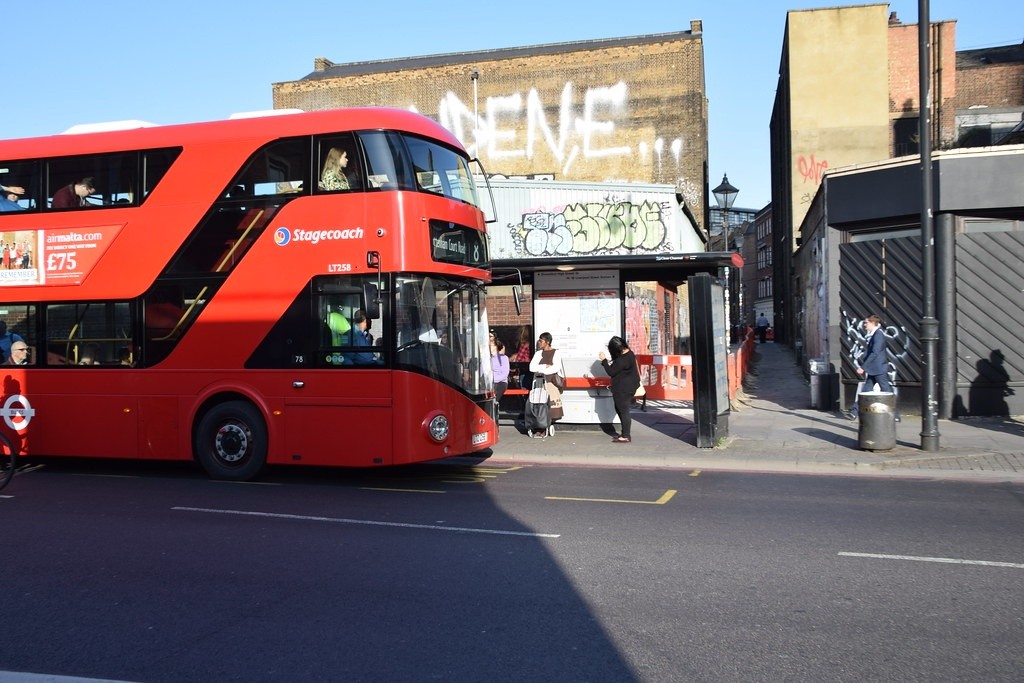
[529,332,564,437]
[0,239,32,269]
[0,341,29,365]
[0,321,24,363]
[78,342,102,366]
[489,329,510,402]
[0,183,26,211]
[342,309,375,365]
[397,304,437,351]
[757,313,768,343]
[840,314,900,421]
[599,336,641,442]
[120,348,130,364]
[516,325,531,402]
[321,147,351,190]
[51,176,129,210]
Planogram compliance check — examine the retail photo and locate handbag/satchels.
[553,374,567,388]
[633,377,646,396]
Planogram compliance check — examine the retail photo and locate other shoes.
[839,409,856,421]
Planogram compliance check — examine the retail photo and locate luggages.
[525,378,555,438]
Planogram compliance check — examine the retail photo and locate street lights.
[711,173,740,354]
[732,231,746,345]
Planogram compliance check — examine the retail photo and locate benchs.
[497,389,529,421]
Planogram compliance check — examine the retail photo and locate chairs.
[326,312,350,365]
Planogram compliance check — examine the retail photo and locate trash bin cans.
[807,359,831,409]
[859,391,897,450]
[796,342,803,366]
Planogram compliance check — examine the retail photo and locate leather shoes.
[613,436,632,443]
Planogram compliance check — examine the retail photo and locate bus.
[0,106,526,481]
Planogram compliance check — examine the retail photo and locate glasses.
[863,321,869,325]
[14,348,29,352]
[489,335,495,338]
